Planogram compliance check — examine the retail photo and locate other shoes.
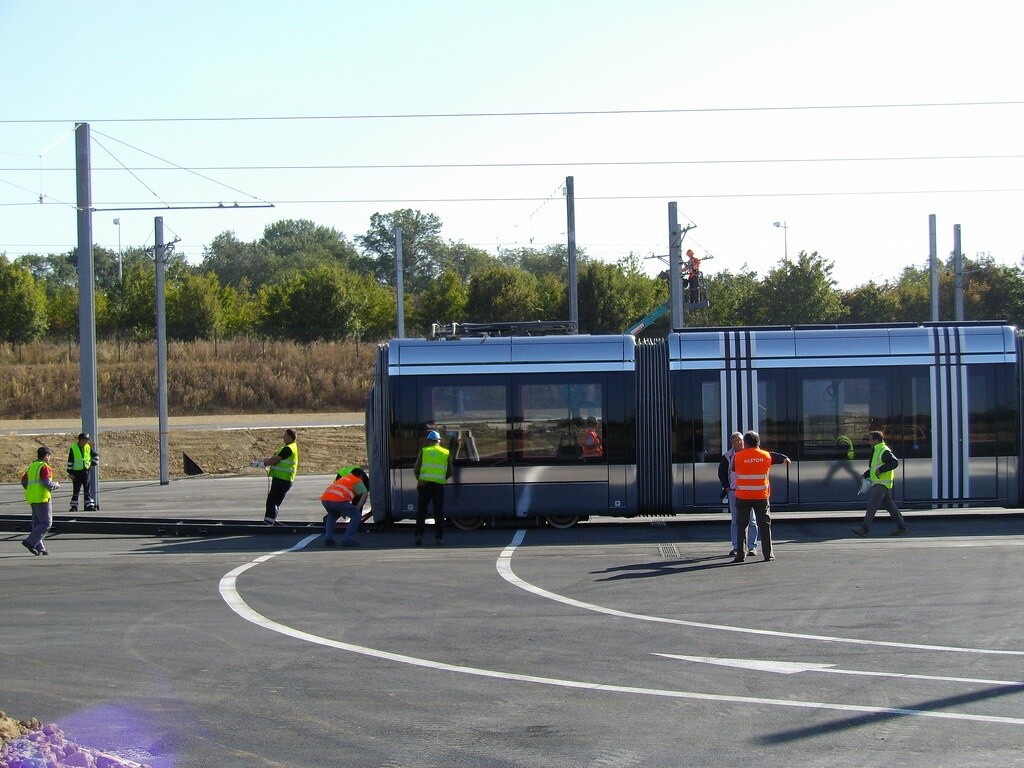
[891,527,910,536]
[414,535,422,544]
[765,556,775,560]
[344,541,359,547]
[733,558,744,562]
[851,527,868,537]
[325,538,334,544]
[436,538,444,545]
[22,541,38,556]
[87,506,97,511]
[730,549,737,555]
[70,506,76,511]
[37,551,48,556]
[749,547,757,555]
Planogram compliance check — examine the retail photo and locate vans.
[881,424,927,443]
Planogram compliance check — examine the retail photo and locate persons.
[682,249,700,303]
[825,426,860,481]
[851,431,910,536]
[67,433,99,512]
[581,416,603,456]
[258,428,298,525]
[718,432,791,556]
[730,430,775,562]
[320,468,368,547]
[21,447,60,556]
[323,465,369,527]
[414,430,454,547]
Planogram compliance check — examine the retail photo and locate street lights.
[773,220,788,285]
[113,218,123,285]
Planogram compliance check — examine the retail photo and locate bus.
[363,320,1024,533]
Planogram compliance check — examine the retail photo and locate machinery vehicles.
[623,270,711,335]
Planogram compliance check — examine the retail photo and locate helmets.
[427,431,442,440]
[858,478,871,495]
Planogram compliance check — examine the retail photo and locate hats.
[79,434,92,440]
